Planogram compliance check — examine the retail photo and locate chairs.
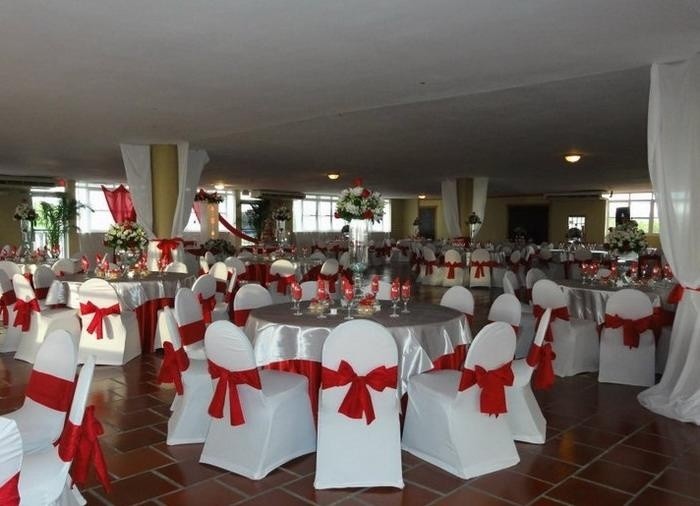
[508,308,551,445]
[1,236,273,366]
[399,322,521,479]
[1,419,25,506]
[199,319,317,481]
[312,320,405,490]
[160,309,214,447]
[20,355,95,506]
[0,329,76,452]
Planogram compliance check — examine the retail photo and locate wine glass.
[579,260,674,286]
[82,256,168,281]
[1,247,61,261]
[289,278,412,320]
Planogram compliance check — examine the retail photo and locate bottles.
[253,246,308,259]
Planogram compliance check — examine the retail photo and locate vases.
[20,219,33,249]
[469,224,476,238]
[348,219,370,271]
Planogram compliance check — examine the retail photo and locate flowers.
[103,220,150,249]
[334,176,386,225]
[466,211,483,224]
[11,199,37,219]
[602,222,650,253]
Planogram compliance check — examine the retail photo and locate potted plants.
[41,199,95,258]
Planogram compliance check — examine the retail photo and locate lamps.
[213,181,225,191]
[328,173,339,179]
[563,154,582,165]
